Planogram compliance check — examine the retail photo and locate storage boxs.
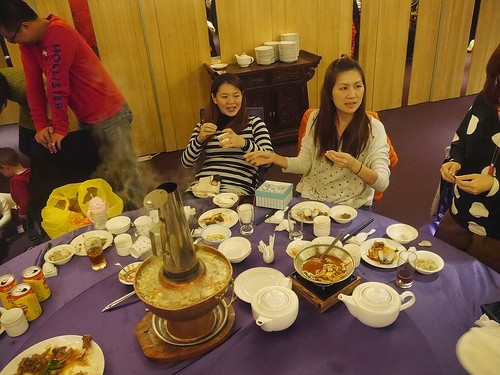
[255,180,293,209]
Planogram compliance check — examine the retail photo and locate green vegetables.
[42,360,65,375]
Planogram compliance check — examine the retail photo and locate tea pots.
[251,278,299,332]
[338,282,416,328]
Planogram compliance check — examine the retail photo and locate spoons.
[355,228,376,242]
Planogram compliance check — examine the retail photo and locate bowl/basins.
[199,224,231,247]
[44,244,75,266]
[386,223,419,244]
[212,193,238,208]
[118,262,143,285]
[106,216,131,235]
[293,236,355,285]
[218,236,252,264]
[408,250,445,274]
[330,205,357,223]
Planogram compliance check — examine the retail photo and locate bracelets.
[356,164,362,175]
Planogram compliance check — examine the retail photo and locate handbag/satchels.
[40,178,124,241]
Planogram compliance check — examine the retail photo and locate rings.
[52,143,55,145]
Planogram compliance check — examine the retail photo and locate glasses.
[6,20,26,44]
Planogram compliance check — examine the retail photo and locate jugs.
[235,53,254,68]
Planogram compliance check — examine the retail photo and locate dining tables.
[0,195,500,375]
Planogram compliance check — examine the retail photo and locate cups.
[313,215,331,237]
[217,132,230,148]
[342,243,361,269]
[287,209,304,241]
[84,215,153,271]
[0,308,30,337]
[236,203,254,235]
[395,250,418,289]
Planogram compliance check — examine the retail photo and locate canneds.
[11,283,42,321]
[21,266,51,302]
[0,274,19,309]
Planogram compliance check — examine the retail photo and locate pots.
[198,208,238,228]
[132,242,232,340]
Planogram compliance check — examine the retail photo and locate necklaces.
[488,137,500,176]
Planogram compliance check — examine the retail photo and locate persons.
[243,54,390,210]
[0,147,42,252]
[435,43,500,274]
[181,73,275,201]
[0,67,47,158]
[0,0,143,212]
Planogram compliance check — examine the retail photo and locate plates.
[359,237,408,269]
[255,33,300,65]
[210,63,228,70]
[234,267,287,303]
[290,201,330,224]
[0,334,105,375]
[70,229,113,256]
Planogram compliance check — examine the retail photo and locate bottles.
[87,197,109,230]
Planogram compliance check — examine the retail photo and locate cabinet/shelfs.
[203,49,322,144]
